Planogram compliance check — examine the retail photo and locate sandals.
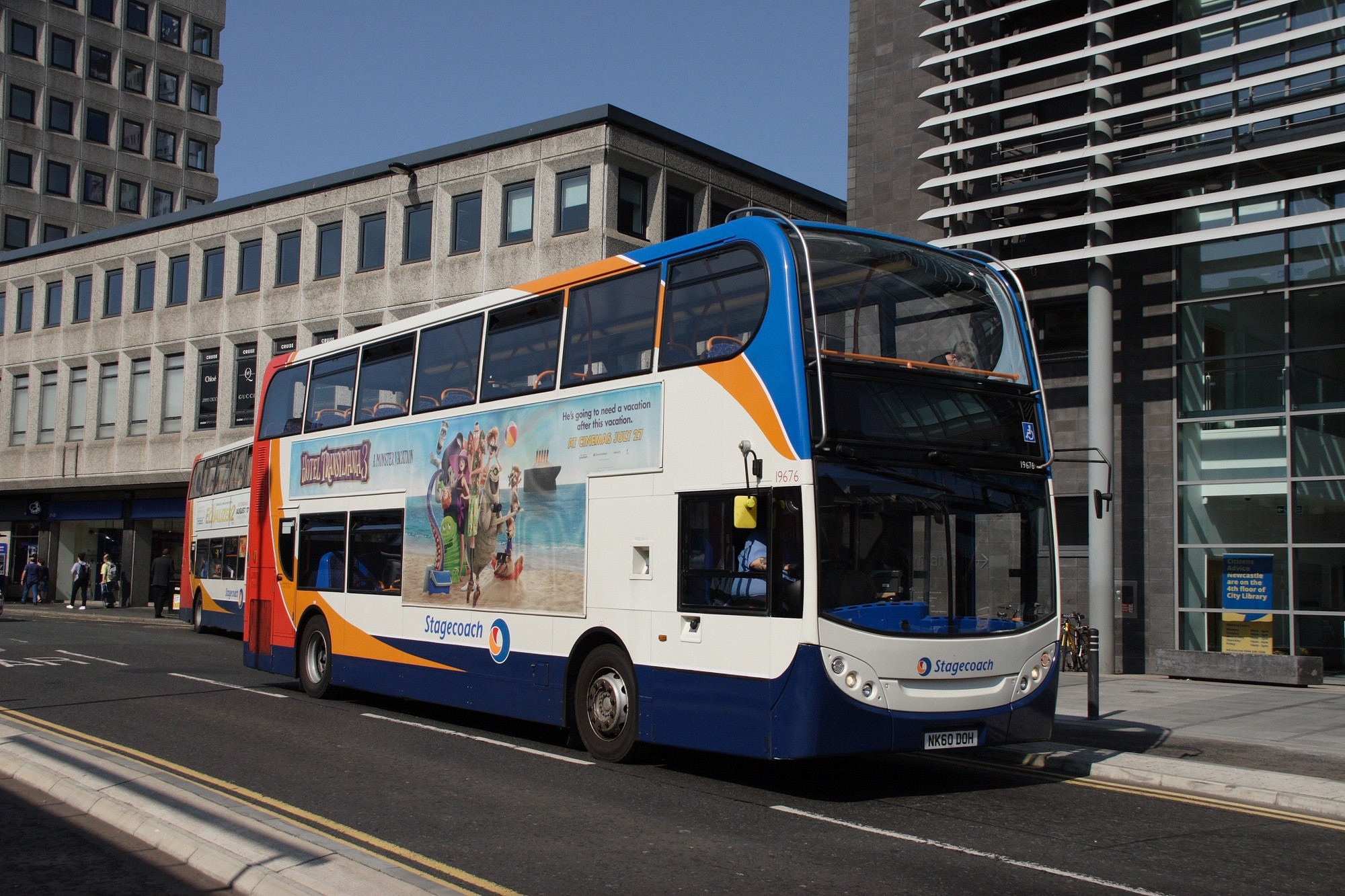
[37,599,42,603]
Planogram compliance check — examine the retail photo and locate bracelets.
[22,580,24,581]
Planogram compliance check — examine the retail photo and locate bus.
[242,203,1115,767]
[180,435,254,634]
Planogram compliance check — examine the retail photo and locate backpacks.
[76,561,91,580]
[105,562,119,580]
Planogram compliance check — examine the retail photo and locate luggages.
[100,582,116,608]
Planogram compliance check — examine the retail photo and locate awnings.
[43,498,124,520]
[0,496,42,521]
[129,497,186,518]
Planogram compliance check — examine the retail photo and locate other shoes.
[34,602,37,605]
[67,605,74,609]
[156,615,164,618]
[79,606,86,610]
[21,602,26,604]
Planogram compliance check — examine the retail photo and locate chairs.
[701,529,727,606]
[192,476,251,496]
[312,544,402,596]
[259,335,746,442]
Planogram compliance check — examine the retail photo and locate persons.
[100,554,116,607]
[66,553,90,610]
[37,560,49,603]
[200,558,234,579]
[731,508,795,617]
[21,557,40,605]
[921,340,980,372]
[150,549,175,617]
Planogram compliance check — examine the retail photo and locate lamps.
[387,161,416,183]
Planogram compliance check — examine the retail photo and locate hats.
[78,553,85,561]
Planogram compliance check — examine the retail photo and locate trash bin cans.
[168,581,180,614]
